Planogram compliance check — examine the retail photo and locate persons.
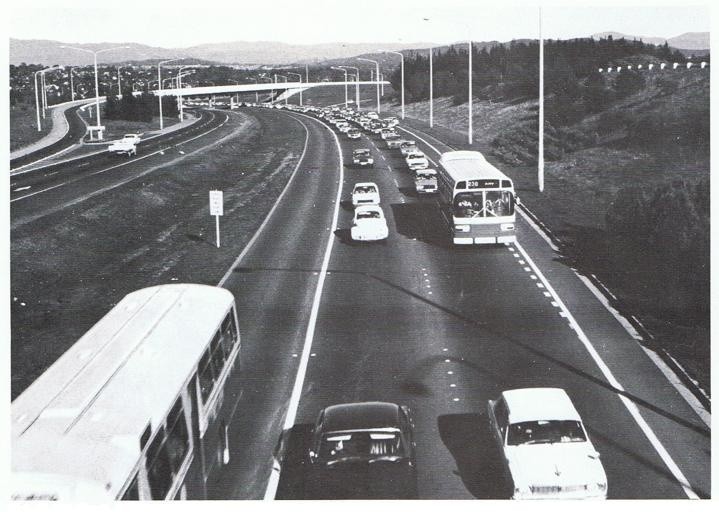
[486,202,492,217]
[373,212,380,218]
[355,187,365,193]
[369,187,375,192]
[458,197,472,210]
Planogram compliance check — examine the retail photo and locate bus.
[12,279,241,497]
[435,149,520,251]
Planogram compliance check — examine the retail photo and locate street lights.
[32,45,200,138]
[203,72,302,106]
[330,37,434,129]
[424,17,474,144]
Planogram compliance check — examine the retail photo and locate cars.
[350,204,389,243]
[108,131,142,158]
[350,180,380,209]
[486,385,611,499]
[301,400,418,498]
[353,147,374,168]
[384,134,440,195]
[185,99,401,140]
